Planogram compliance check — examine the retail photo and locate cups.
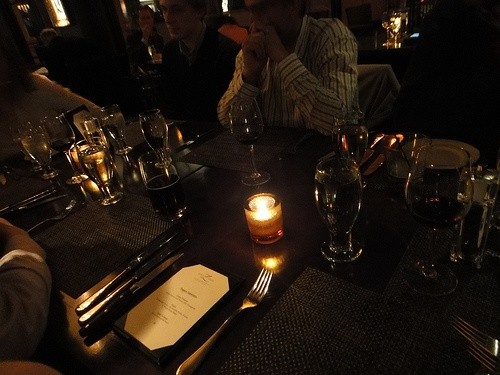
[145,173,184,218]
[382,132,432,195]
[460,163,499,269]
[242,191,285,245]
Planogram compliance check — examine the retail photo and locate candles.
[246,196,281,236]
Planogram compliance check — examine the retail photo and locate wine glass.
[9,104,187,222]
[312,158,364,262]
[380,8,409,49]
[403,144,474,296]
[228,96,271,186]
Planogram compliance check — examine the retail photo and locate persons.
[0,0,247,375]
[218,0,360,138]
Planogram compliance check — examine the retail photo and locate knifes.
[363,133,404,176]
[359,133,384,169]
[0,187,69,213]
[72,225,194,337]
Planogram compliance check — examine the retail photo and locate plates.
[403,138,480,170]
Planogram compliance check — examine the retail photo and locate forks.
[26,199,76,232]
[175,268,274,375]
[451,315,500,375]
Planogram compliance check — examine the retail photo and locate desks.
[0,116,500,375]
